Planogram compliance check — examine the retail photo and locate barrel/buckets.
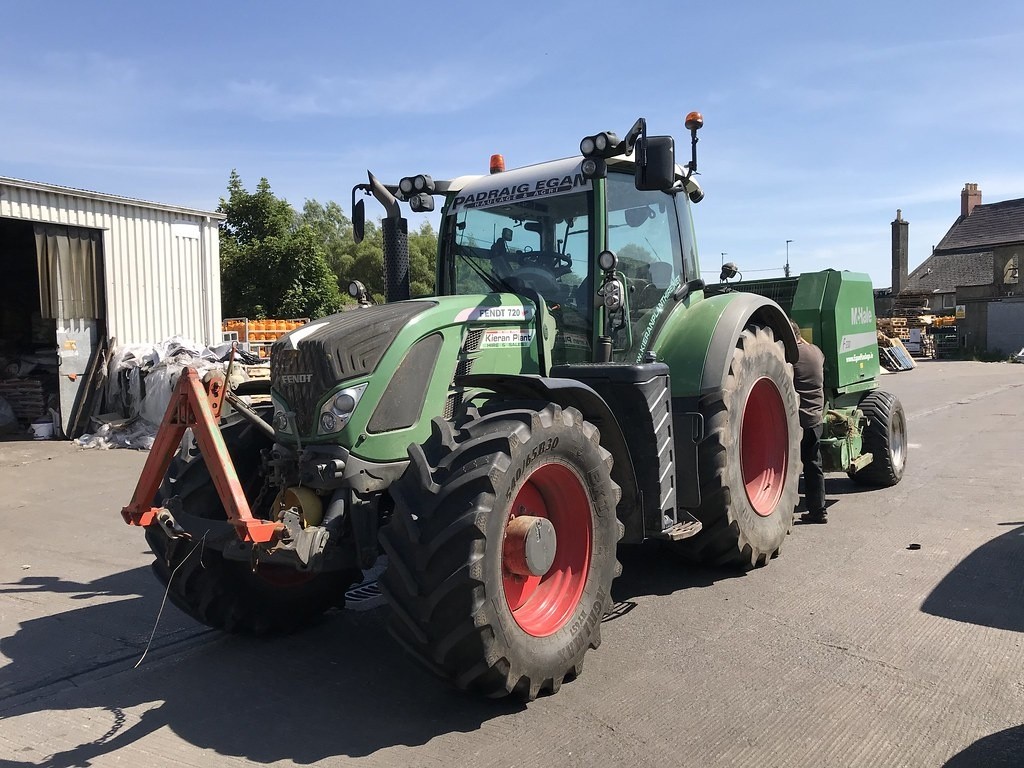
[31,421,54,440]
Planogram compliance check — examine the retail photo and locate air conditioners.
[223,330,239,343]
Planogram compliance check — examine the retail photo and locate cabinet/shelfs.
[934,326,959,359]
[223,318,311,361]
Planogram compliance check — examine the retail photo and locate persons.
[788,318,829,524]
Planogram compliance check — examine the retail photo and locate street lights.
[721,252,727,283]
[784,240,794,277]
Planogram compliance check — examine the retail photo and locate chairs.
[577,256,651,311]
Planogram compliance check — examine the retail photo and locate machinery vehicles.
[119,109,908,705]
